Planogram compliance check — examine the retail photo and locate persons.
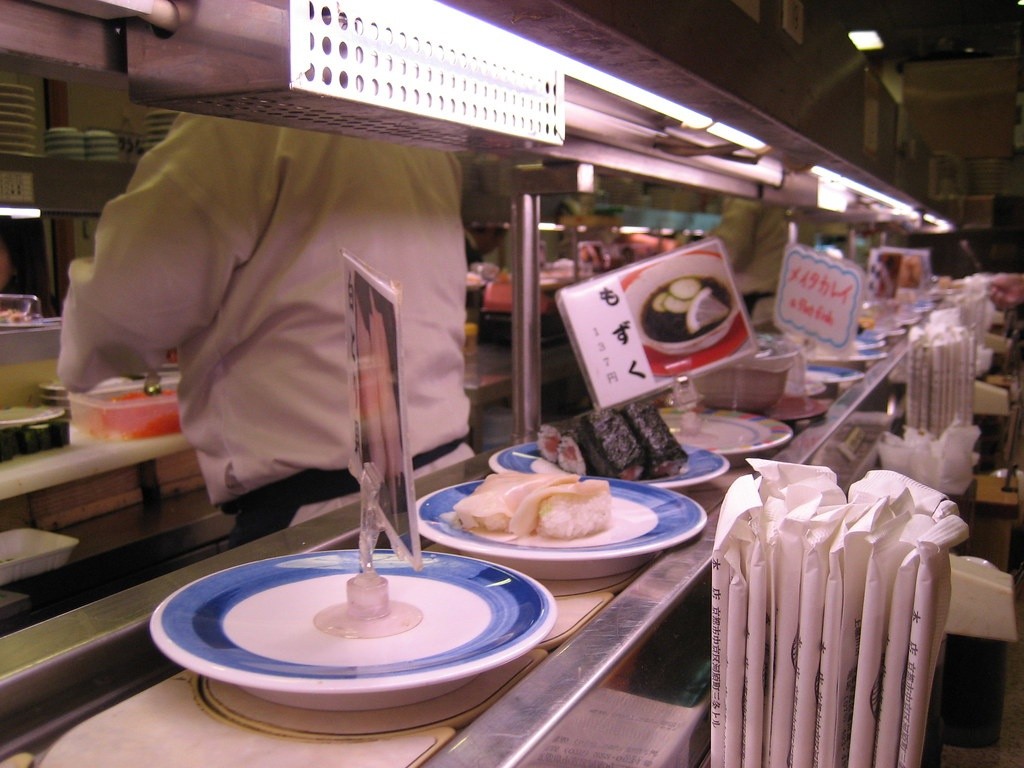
[0,216,58,319]
[627,195,816,317]
[986,272,1024,311]
[56,113,477,550]
[462,222,511,272]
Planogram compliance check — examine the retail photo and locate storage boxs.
[66,378,184,448]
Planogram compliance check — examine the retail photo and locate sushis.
[536,398,690,481]
[452,474,613,541]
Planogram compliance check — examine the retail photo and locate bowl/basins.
[623,253,739,355]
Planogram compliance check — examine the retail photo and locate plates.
[488,441,731,489]
[45,127,84,160]
[0,528,78,585]
[0,83,36,157]
[415,477,708,579]
[784,365,866,396]
[150,550,557,711]
[84,130,118,161]
[0,376,127,430]
[143,109,178,152]
[659,407,793,454]
[682,400,828,424]
[805,295,941,364]
[605,176,720,215]
